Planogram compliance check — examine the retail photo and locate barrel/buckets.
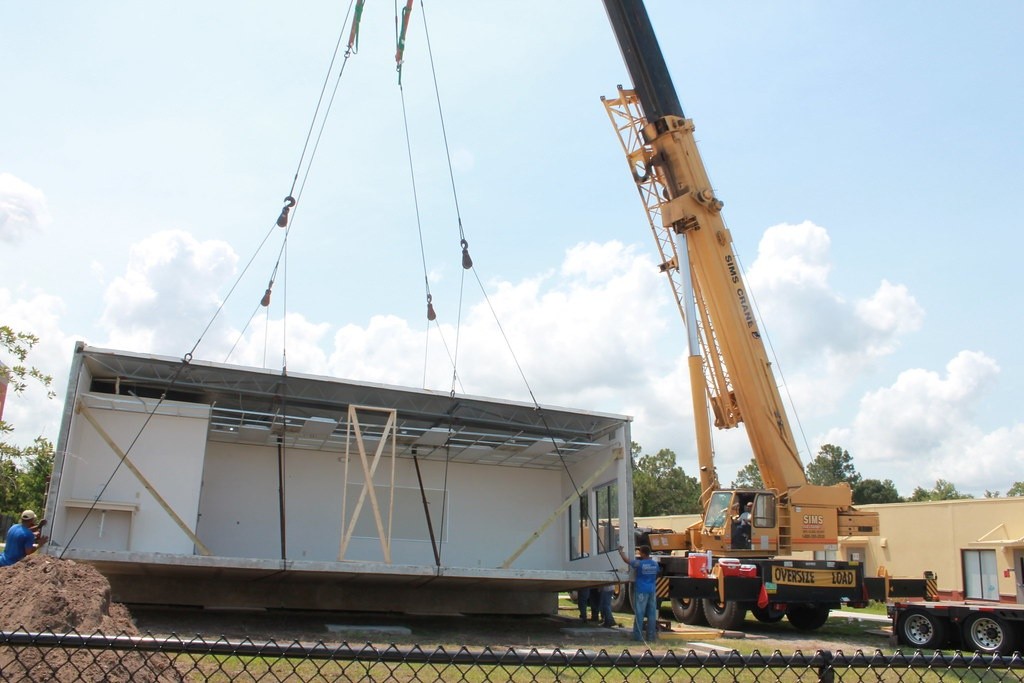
[739,563,757,578]
[718,558,741,577]
[687,553,708,578]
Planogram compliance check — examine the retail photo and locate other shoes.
[599,618,616,627]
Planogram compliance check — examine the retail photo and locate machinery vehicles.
[570,0,880,633]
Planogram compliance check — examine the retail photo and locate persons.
[601,585,617,627]
[0,510,50,567]
[617,545,659,642]
[579,585,600,624]
[731,502,755,542]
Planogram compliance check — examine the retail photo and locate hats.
[21,509,36,520]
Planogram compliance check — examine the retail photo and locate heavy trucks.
[885,599,1024,656]
